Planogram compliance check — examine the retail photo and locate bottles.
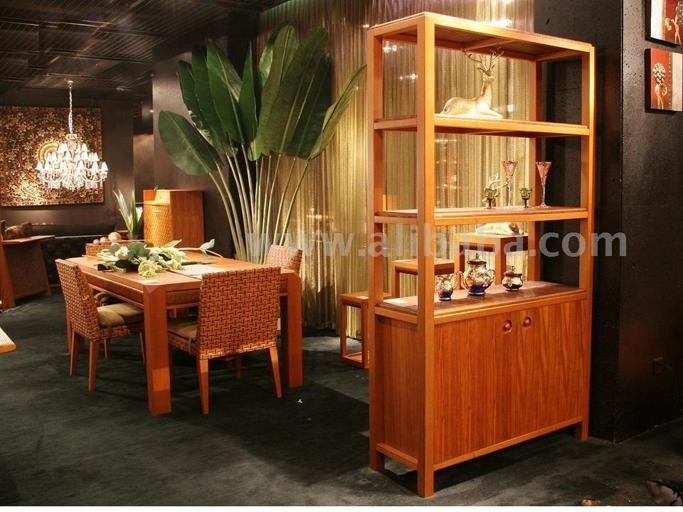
[436,273,454,301]
[501,266,524,291]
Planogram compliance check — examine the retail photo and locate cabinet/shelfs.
[338,232,529,369]
[142,188,207,251]
[362,9,595,498]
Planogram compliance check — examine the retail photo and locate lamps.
[34,78,109,191]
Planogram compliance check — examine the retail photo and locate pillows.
[4,221,34,240]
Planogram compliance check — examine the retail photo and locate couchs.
[32,222,115,237]
[40,234,125,288]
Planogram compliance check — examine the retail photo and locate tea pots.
[455,253,496,296]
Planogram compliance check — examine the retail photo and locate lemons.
[93,231,122,244]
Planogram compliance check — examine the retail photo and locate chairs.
[225,244,303,368]
[85,243,133,308]
[55,258,145,393]
[167,265,283,415]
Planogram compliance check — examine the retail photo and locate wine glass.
[483,187,497,210]
[533,161,552,210]
[518,187,533,208]
[501,159,518,208]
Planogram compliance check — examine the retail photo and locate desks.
[1,235,56,308]
[63,250,304,416]
